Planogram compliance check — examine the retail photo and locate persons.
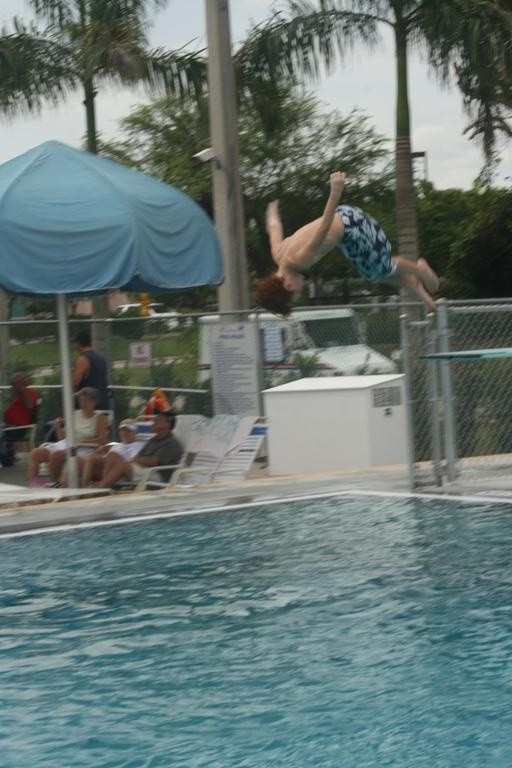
[86,411,182,498]
[69,333,139,409]
[253,167,438,316]
[59,418,146,488]
[0,372,39,467]
[27,388,109,485]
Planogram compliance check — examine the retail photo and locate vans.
[196,309,396,392]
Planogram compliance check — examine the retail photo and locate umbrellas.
[0,140,227,484]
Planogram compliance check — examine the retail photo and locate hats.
[118,419,138,431]
[72,387,100,402]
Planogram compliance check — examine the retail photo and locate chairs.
[0,397,255,499]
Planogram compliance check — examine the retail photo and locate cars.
[112,304,193,338]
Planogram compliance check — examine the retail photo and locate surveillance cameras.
[192,147,217,163]
